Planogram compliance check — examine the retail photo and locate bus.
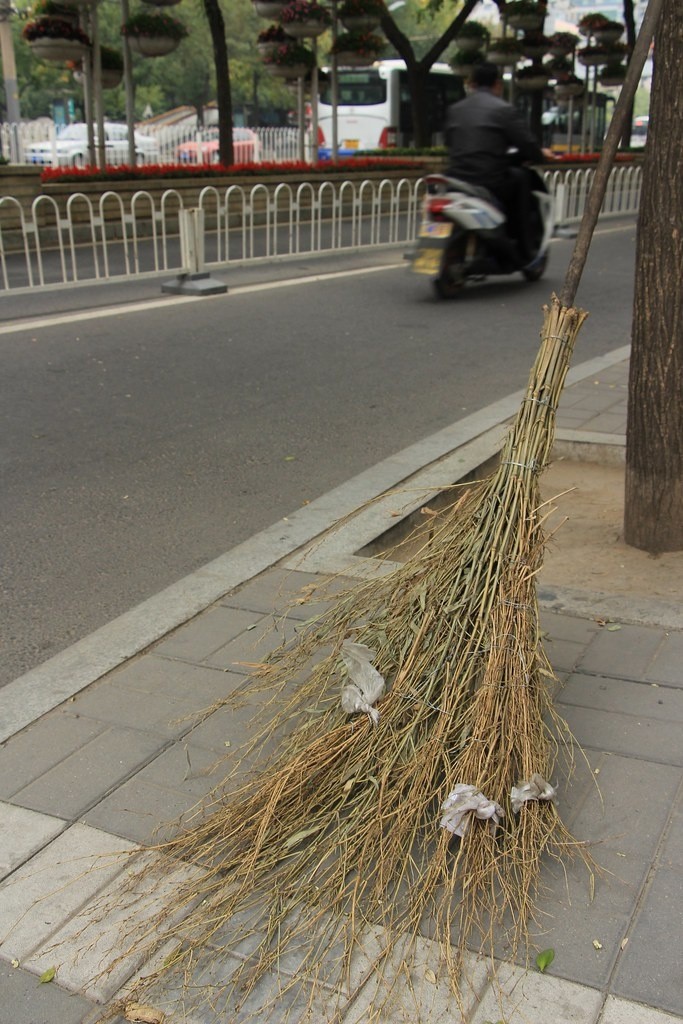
[299,57,620,166]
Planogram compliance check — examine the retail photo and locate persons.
[439,61,558,260]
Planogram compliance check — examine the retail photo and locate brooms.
[1,0,667,1024]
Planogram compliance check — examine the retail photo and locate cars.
[22,119,164,172]
[172,126,265,169]
[625,114,649,147]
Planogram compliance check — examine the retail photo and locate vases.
[250,0,384,102]
[448,0,631,109]
[20,0,189,89]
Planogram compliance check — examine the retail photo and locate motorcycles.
[400,146,567,301]
